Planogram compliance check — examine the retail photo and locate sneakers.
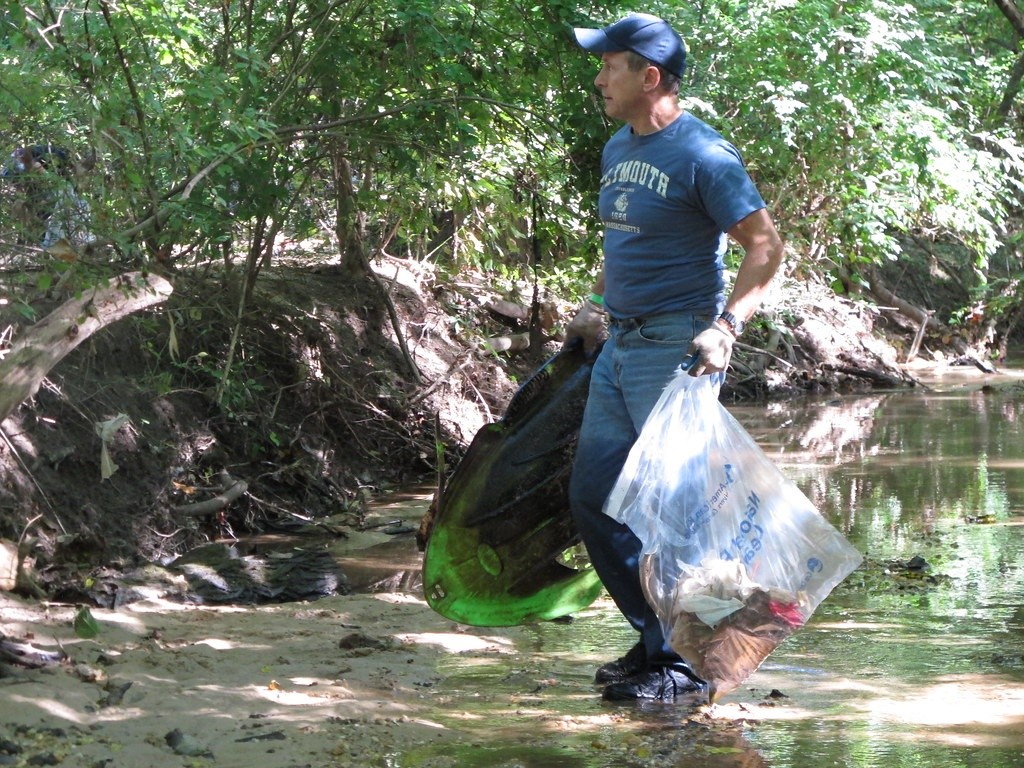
[595,647,650,683]
[603,664,707,701]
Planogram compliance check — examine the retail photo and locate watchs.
[720,309,748,336]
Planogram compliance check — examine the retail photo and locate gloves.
[564,301,606,358]
[686,320,736,377]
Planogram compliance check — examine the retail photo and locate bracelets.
[584,300,610,316]
[711,318,737,344]
[588,291,607,306]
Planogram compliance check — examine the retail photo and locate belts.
[608,313,714,329]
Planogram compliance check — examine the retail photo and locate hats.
[574,13,687,79]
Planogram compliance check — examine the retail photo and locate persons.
[562,11,790,704]
